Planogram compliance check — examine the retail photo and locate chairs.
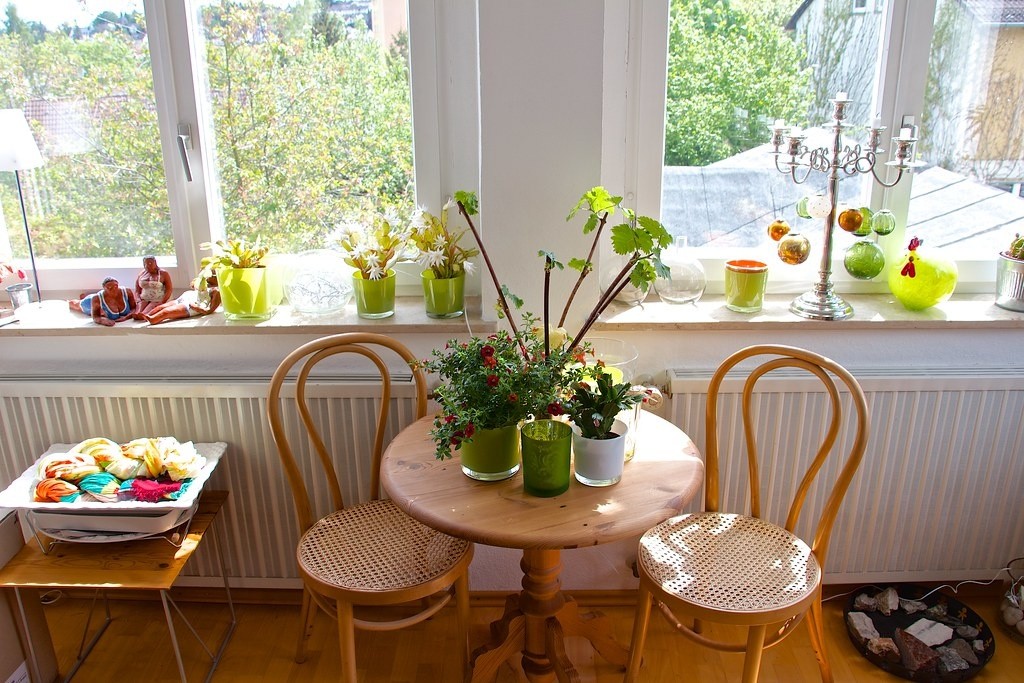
[266,330,475,683]
[623,343,872,683]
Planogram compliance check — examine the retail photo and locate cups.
[5,283,33,309]
[521,419,573,497]
[724,258,768,313]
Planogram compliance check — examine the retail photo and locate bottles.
[653,234,705,304]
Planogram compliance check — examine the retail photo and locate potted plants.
[189,235,283,322]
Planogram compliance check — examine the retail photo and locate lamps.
[0,108,70,329]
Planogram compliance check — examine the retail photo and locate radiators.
[665,364,1024,584]
[0,370,418,591]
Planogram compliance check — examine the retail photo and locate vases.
[994,251,1024,314]
[520,420,573,498]
[420,269,466,319]
[459,424,521,483]
[284,249,354,317]
[351,268,397,320]
[571,419,629,488]
[653,235,707,309]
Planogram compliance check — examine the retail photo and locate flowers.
[406,311,607,462]
[562,373,642,440]
[1004,232,1024,259]
[406,197,481,279]
[324,205,412,282]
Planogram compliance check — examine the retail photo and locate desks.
[379,407,705,683]
[0,489,239,683]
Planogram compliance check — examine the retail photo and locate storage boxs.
[0,507,60,683]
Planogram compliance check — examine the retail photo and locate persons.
[137,268,221,325]
[68,276,136,327]
[132,255,172,322]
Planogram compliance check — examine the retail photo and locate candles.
[835,88,847,101]
[790,122,801,137]
[775,118,785,128]
[899,128,911,140]
[870,112,881,129]
